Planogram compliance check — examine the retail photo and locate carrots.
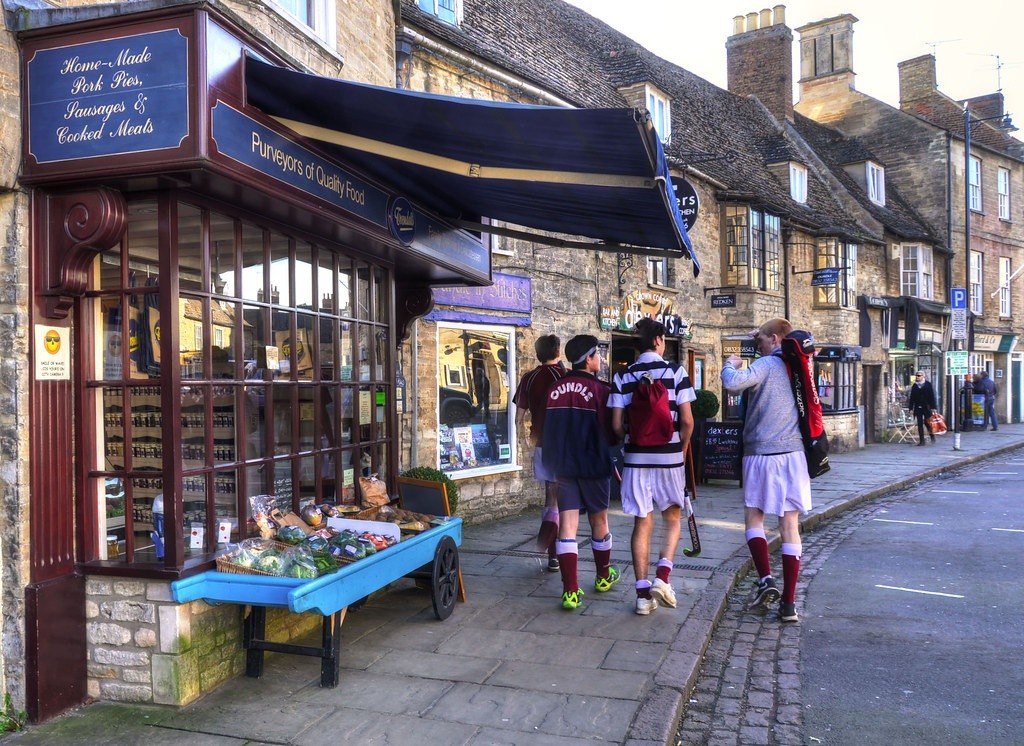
[362,533,388,550]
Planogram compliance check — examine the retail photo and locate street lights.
[963,108,1011,432]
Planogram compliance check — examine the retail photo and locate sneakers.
[561,586,585,609]
[649,577,677,608]
[594,564,621,592]
[548,557,560,572]
[779,596,798,621]
[748,576,780,615]
[635,595,660,615]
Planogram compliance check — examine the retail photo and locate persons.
[972,371,998,431]
[909,371,937,447]
[719,318,812,623]
[474,368,495,415]
[512,318,697,616]
[182,330,387,513]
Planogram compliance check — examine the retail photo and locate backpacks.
[626,364,677,447]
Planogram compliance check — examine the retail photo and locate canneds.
[107,535,118,559]
[105,384,234,527]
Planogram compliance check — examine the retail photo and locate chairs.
[888,405,918,444]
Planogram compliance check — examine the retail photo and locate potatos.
[376,508,434,530]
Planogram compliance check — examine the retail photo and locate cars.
[439,386,474,428]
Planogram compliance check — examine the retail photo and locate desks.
[170,515,462,690]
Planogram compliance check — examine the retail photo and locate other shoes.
[930,436,935,445]
[917,439,925,446]
[979,426,986,431]
[990,426,998,431]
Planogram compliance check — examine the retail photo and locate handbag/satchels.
[257,508,316,540]
[926,410,947,435]
[360,472,391,508]
[772,330,830,479]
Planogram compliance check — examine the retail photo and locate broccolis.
[276,525,376,560]
[231,549,315,578]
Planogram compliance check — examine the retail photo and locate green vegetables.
[309,551,340,575]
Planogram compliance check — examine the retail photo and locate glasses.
[915,375,923,377]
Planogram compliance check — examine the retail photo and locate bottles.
[150,494,165,561]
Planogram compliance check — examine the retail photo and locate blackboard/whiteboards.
[697,422,744,479]
[394,475,467,603]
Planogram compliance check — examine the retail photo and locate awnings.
[244,49,696,258]
[450,326,683,373]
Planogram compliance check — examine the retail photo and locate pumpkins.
[301,503,323,526]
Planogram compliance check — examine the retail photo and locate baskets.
[217,537,359,577]
[357,505,426,535]
